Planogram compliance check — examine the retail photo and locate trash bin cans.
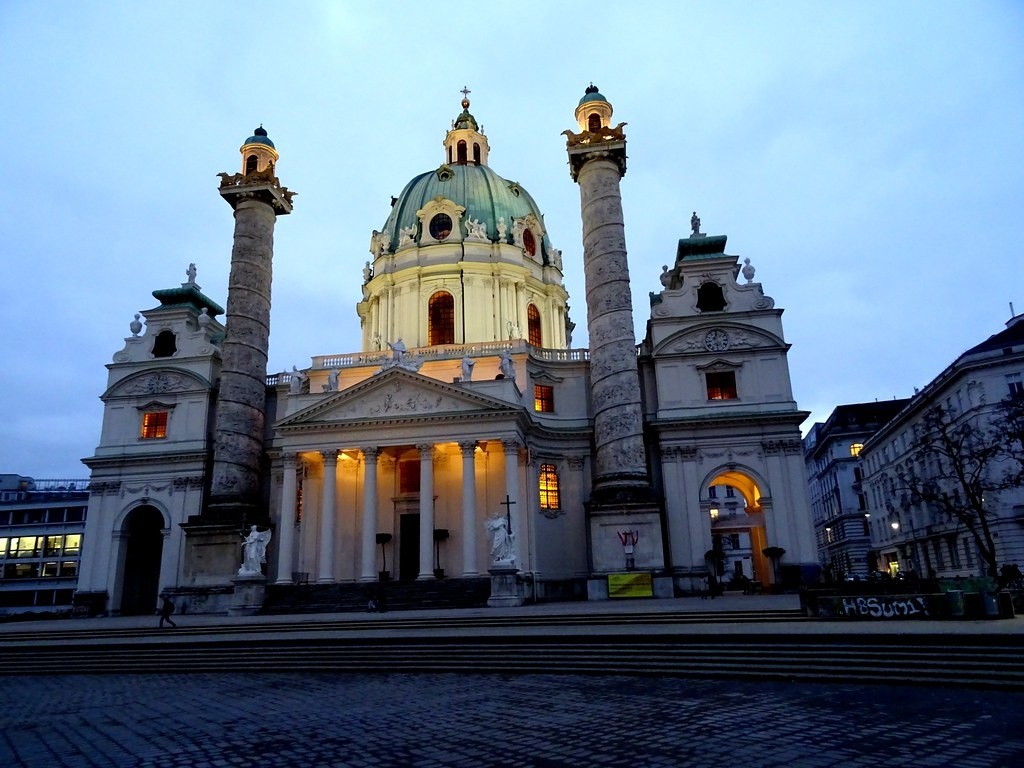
[980,589,999,616]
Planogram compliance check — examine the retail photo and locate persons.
[986,564,1022,597]
[740,574,750,595]
[159,597,176,629]
[385,336,407,363]
[363,215,556,282]
[240,524,262,572]
[368,594,388,613]
[488,512,511,561]
[284,365,304,393]
[457,354,477,381]
[700,574,718,600]
[327,366,342,389]
[498,349,517,378]
